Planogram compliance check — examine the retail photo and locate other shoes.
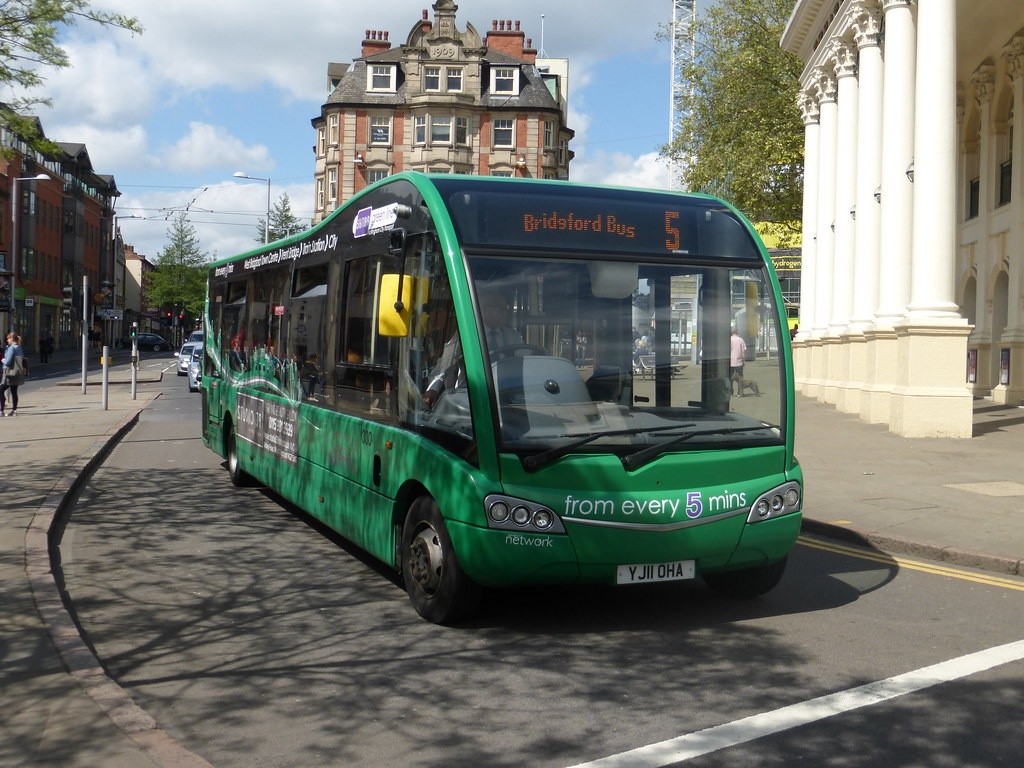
[0,411,5,417]
[8,411,17,416]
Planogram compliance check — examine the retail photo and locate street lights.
[10,174,52,333]
[233,171,271,244]
[112,215,143,350]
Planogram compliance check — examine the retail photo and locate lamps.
[830,219,834,232]
[849,204,855,220]
[873,184,881,204]
[516,153,526,168]
[353,151,364,167]
[905,153,914,183]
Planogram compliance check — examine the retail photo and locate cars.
[174,341,197,376]
[184,345,203,393]
[115,332,174,353]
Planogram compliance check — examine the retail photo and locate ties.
[488,331,497,350]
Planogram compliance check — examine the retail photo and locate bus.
[200,169,806,630]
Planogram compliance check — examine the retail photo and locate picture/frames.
[968,349,977,383]
[1001,348,1010,385]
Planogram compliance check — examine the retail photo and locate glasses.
[486,304,511,312]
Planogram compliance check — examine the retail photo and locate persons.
[730,327,747,398]
[790,324,799,341]
[423,290,535,413]
[631,326,656,364]
[40,333,54,363]
[88,327,94,349]
[0,330,24,417]
[95,329,102,354]
[576,329,587,368]
[229,336,330,402]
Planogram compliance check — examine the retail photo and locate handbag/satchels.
[1,347,25,386]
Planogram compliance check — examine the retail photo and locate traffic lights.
[62,286,79,321]
[166,310,172,322]
[178,314,184,326]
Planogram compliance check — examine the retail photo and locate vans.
[184,330,204,343]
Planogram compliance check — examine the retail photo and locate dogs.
[731,372,760,398]
